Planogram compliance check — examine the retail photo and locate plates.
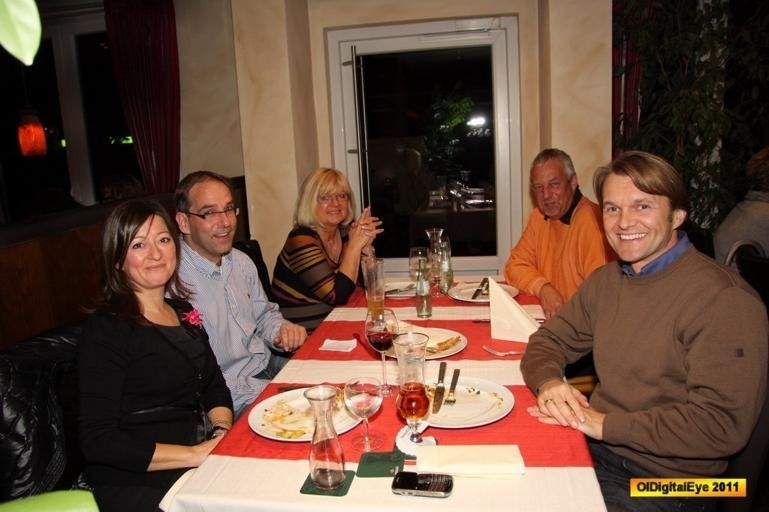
[248,281,519,442]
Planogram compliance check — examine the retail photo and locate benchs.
[0,240,270,512]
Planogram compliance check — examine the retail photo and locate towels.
[489,275,540,344]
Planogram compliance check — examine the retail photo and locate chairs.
[725,239,769,272]
[565,228,715,394]
[714,272,769,512]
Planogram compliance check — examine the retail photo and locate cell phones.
[391,470,454,498]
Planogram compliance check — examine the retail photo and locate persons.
[269,168,384,337]
[504,147,620,320]
[67,197,234,511]
[519,153,769,512]
[713,147,769,306]
[164,170,308,421]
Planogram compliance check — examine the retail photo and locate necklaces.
[329,251,339,264]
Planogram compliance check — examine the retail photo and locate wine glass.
[344,229,453,451]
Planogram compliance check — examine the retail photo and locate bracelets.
[207,426,230,440]
[212,420,232,430]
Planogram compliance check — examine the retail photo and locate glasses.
[178,208,240,220]
[318,193,349,203]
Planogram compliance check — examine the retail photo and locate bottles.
[302,383,348,490]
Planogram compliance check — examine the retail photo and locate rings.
[544,398,553,405]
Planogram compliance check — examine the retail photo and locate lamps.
[16,64,50,159]
